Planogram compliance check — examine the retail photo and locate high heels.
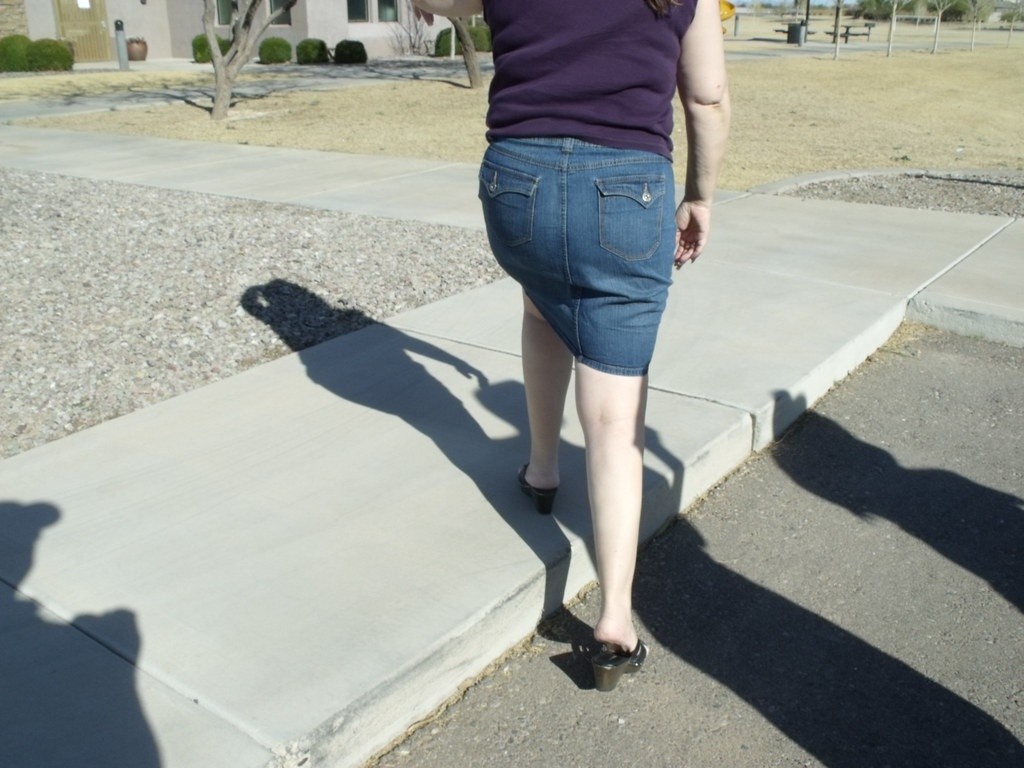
[516,462,559,514]
[592,638,650,692]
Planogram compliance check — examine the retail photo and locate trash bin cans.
[787,23,801,43]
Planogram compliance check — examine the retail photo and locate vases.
[128,39,148,60]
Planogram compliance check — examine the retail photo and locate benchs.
[826,22,874,42]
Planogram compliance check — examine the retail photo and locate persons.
[417,0,731,692]
